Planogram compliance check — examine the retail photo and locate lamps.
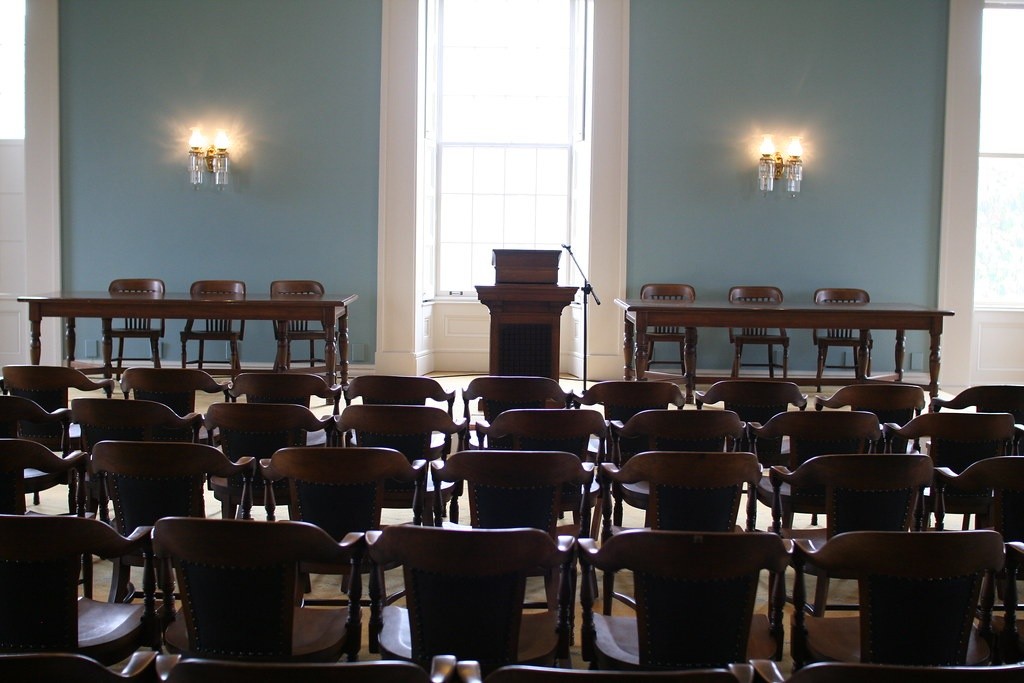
[759,133,804,200]
[186,127,231,193]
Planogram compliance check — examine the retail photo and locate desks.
[17,290,359,391]
[614,298,955,413]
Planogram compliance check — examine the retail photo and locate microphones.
[561,245,572,249]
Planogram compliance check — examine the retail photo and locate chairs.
[180,281,246,370]
[109,279,166,380]
[728,286,790,379]
[271,281,340,367]
[640,283,696,375]
[0,365,1024,683]
[812,288,873,378]
